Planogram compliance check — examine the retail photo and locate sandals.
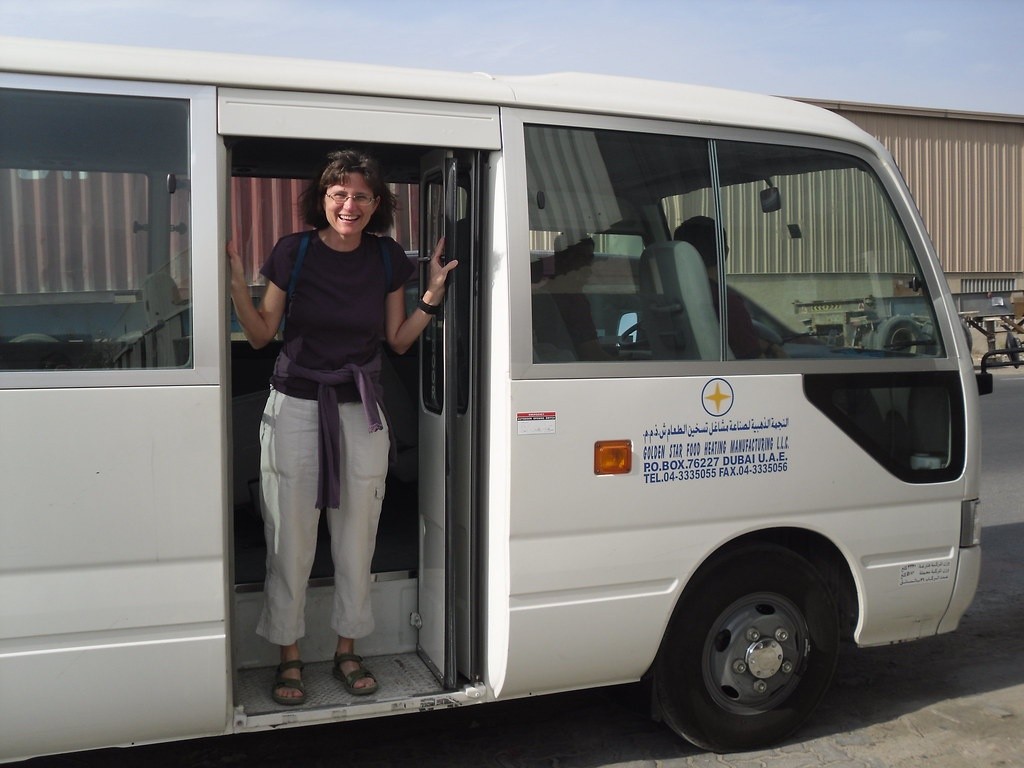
[331,651,377,695]
[271,660,307,705]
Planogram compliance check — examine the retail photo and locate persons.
[547,214,791,359]
[229,151,457,703]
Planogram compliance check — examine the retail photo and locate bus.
[0,32,1024,756]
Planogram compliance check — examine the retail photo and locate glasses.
[325,193,376,206]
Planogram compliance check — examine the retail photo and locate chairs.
[635,249,735,362]
[142,271,182,341]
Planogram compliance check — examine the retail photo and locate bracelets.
[766,342,773,354]
[417,296,440,315]
